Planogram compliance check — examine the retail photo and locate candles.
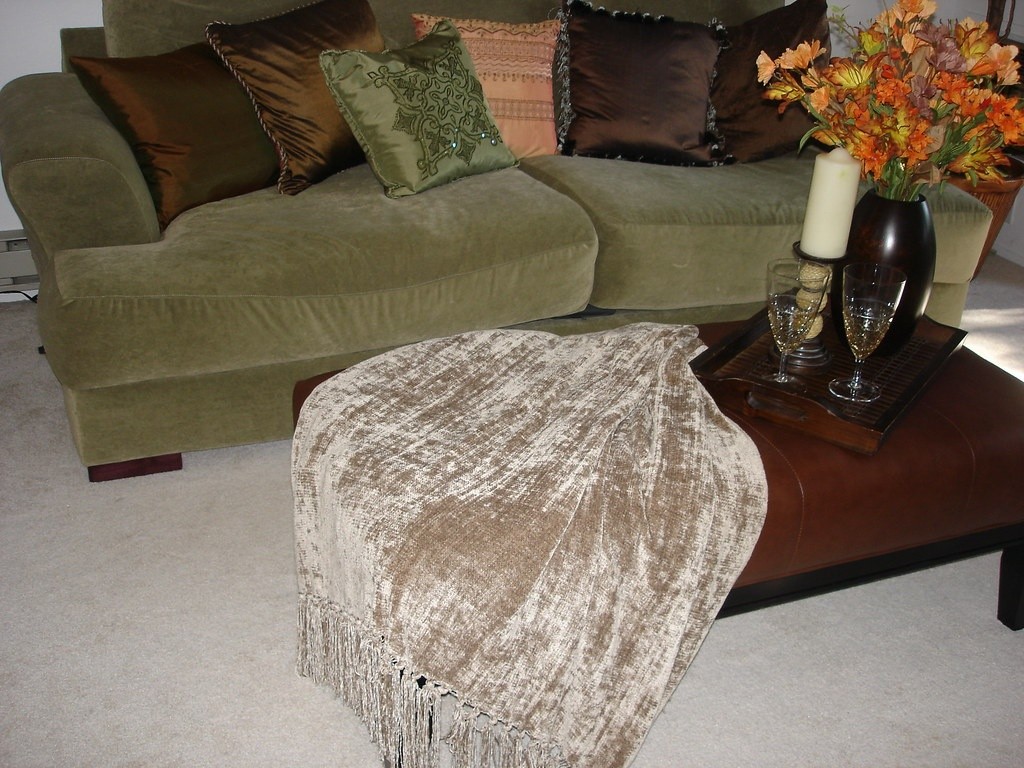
[799,147,861,258]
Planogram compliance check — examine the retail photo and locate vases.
[830,189,936,356]
[948,154,1024,283]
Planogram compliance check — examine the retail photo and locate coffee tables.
[292,315,1023,768]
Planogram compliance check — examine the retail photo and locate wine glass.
[829,263,907,403]
[762,258,833,385]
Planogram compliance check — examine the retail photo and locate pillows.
[412,13,560,160]
[205,0,386,195]
[69,40,281,233]
[711,0,831,164]
[552,0,735,168]
[320,18,521,199]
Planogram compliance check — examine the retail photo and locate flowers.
[756,0,1024,202]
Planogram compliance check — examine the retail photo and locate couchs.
[0,0,994,485]
